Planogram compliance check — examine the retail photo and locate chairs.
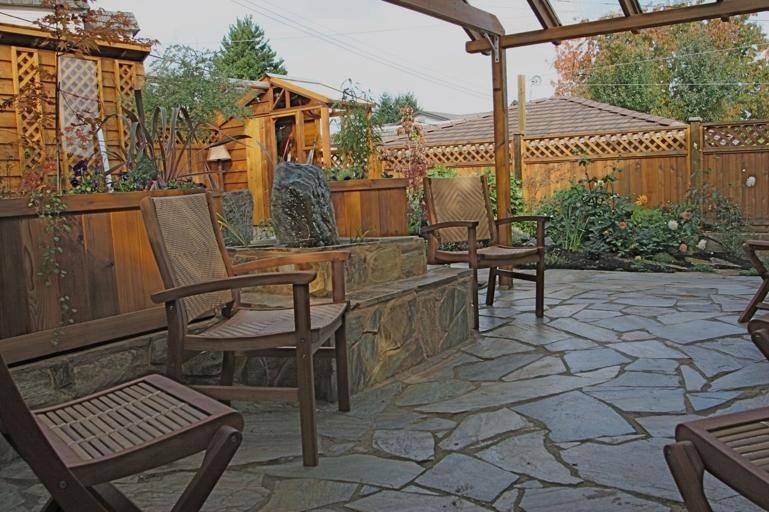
[419,175,553,330]
[1,355,245,512]
[140,190,352,467]
[738,239,769,323]
[747,311,769,360]
[662,404,769,512]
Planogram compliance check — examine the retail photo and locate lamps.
[205,143,233,193]
